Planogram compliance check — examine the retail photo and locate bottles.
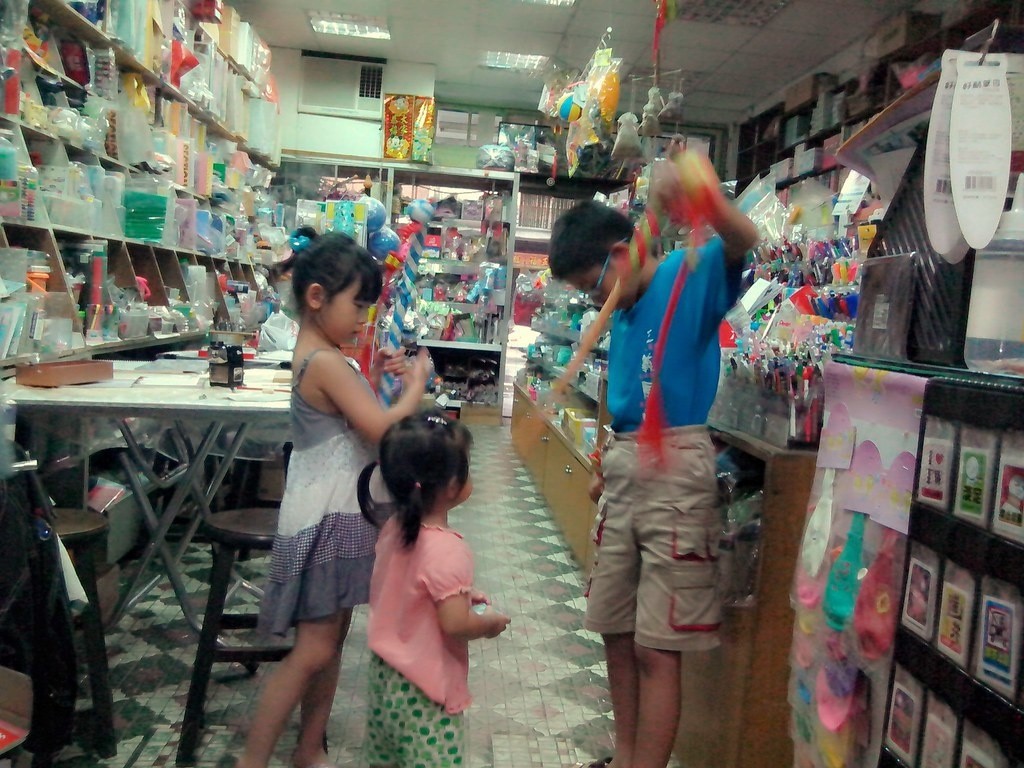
[208,334,244,386]
[495,267,505,289]
[466,280,481,303]
[209,341,230,387]
[964,172,1024,378]
[481,267,495,292]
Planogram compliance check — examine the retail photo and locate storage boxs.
[964,210,1024,377]
[771,8,942,205]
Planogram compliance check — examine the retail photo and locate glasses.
[577,237,629,298]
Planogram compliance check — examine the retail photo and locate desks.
[1,360,293,680]
[159,351,294,370]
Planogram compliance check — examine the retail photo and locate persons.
[239,225,432,768]
[548,154,757,768]
[356,410,509,768]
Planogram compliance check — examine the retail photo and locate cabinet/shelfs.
[773,0,1024,206]
[0,0,281,367]
[280,155,519,407]
[735,101,786,198]
[510,171,1024,768]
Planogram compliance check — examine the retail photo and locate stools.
[52,507,118,758]
[175,508,328,767]
